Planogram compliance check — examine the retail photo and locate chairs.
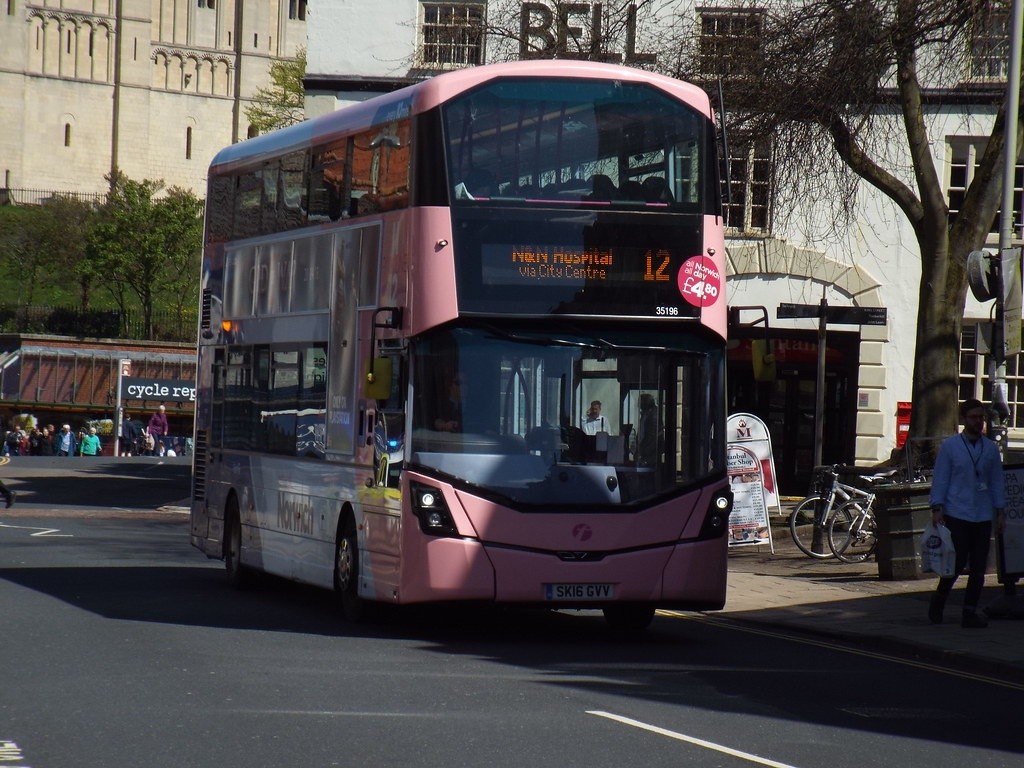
[231,171,679,235]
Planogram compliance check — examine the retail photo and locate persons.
[357,194,380,216]
[582,400,612,436]
[0,480,17,509]
[632,394,665,468]
[3,403,189,459]
[928,398,1008,628]
[427,364,501,436]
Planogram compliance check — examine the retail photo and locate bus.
[189,59,736,623]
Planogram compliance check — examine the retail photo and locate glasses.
[964,414,985,418]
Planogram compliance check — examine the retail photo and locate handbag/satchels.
[920,521,956,578]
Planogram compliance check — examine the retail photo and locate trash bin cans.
[871,483,934,581]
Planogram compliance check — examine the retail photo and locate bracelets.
[932,508,941,513]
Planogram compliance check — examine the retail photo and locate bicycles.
[827,470,933,564]
[790,464,882,559]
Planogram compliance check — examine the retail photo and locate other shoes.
[929,594,989,628]
[121,452,133,458]
[4,492,18,508]
